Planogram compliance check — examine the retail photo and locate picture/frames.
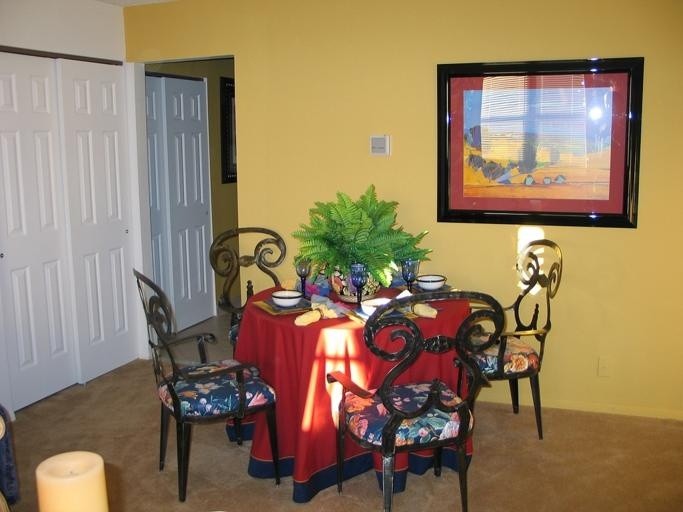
[218,76,236,186]
[435,55,646,231]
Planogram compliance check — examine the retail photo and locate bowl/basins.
[416,274,447,291]
[271,290,303,307]
[360,297,397,317]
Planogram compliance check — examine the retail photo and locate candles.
[33,449,110,512]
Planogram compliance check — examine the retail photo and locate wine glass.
[294,255,311,298]
[400,258,420,293]
[351,265,366,306]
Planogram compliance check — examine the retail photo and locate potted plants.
[289,183,434,302]
